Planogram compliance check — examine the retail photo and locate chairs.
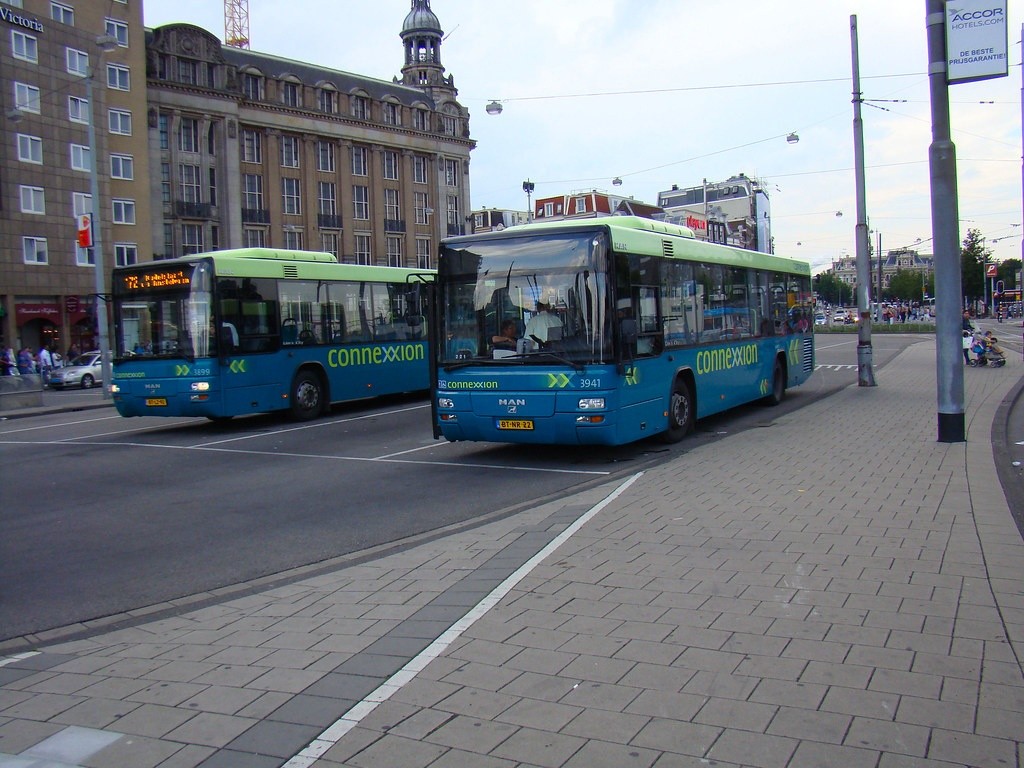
[729,288,746,307]
[750,287,765,306]
[770,285,784,305]
[282,318,298,345]
[299,330,319,345]
[711,288,727,308]
[788,285,804,300]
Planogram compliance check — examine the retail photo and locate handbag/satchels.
[962,330,973,349]
[43,370,50,381]
[888,314,894,318]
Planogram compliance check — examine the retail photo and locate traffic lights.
[996,281,1005,294]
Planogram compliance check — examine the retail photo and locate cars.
[48,349,137,391]
[813,298,880,327]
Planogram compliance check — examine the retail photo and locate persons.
[983,331,996,352]
[84,342,100,353]
[881,302,932,325]
[250,316,268,342]
[844,311,848,321]
[447,330,456,340]
[848,310,852,320]
[690,328,694,338]
[134,340,152,354]
[797,307,809,333]
[0,343,81,385]
[962,310,982,365]
[487,319,518,351]
[1008,305,1016,318]
[792,308,802,332]
[619,302,642,336]
[973,329,985,345]
[524,297,563,349]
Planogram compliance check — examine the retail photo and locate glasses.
[965,313,969,314]
[508,326,513,329]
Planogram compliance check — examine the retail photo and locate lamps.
[797,241,801,245]
[787,131,800,144]
[916,238,921,242]
[612,177,622,186]
[1009,243,1014,247]
[993,239,997,243]
[425,207,434,215]
[835,210,842,217]
[485,100,504,115]
[95,32,118,50]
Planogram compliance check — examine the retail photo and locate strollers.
[966,329,1007,368]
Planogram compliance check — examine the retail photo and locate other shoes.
[44,384,48,387]
[966,362,970,366]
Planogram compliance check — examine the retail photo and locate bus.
[77,248,439,423]
[404,215,815,452]
